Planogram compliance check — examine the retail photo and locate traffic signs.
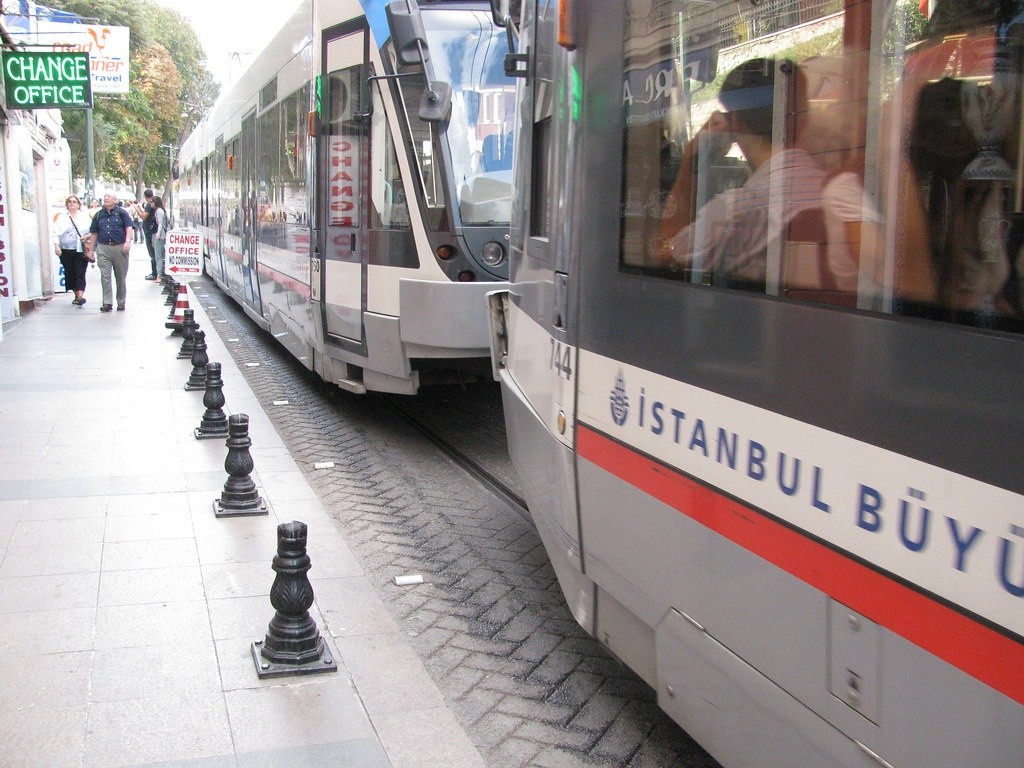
[164,228,204,277]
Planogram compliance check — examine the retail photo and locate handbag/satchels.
[82,243,95,263]
[132,218,138,229]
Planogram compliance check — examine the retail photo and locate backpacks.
[163,212,173,235]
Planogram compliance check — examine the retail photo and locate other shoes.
[145,274,156,280]
[134,241,137,244]
[139,241,144,244]
[78,298,86,306]
[100,304,113,311]
[117,305,125,310]
[72,296,79,304]
[155,280,161,283]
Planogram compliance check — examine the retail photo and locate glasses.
[150,201,154,203]
[68,200,77,203]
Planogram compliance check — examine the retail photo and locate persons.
[645,57,1023,311]
[52,188,169,312]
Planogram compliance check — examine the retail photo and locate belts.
[98,241,118,247]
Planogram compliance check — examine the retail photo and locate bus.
[455,0,1024,768]
[170,0,575,396]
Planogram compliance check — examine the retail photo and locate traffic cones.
[171,279,191,324]
